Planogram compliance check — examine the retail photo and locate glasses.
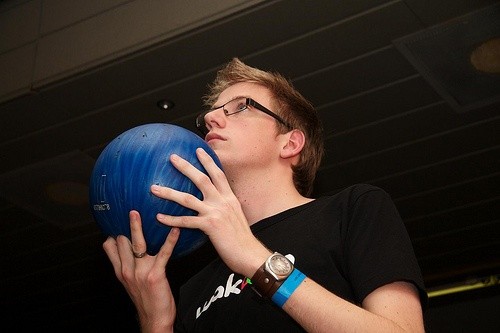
[194,97,294,134]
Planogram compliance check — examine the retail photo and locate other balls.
[89,123,224,257]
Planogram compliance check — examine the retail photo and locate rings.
[131,249,147,259]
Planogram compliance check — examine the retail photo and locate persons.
[103,57,427,333]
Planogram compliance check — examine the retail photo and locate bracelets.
[270,268,307,307]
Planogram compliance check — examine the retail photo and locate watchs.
[248,251,294,299]
